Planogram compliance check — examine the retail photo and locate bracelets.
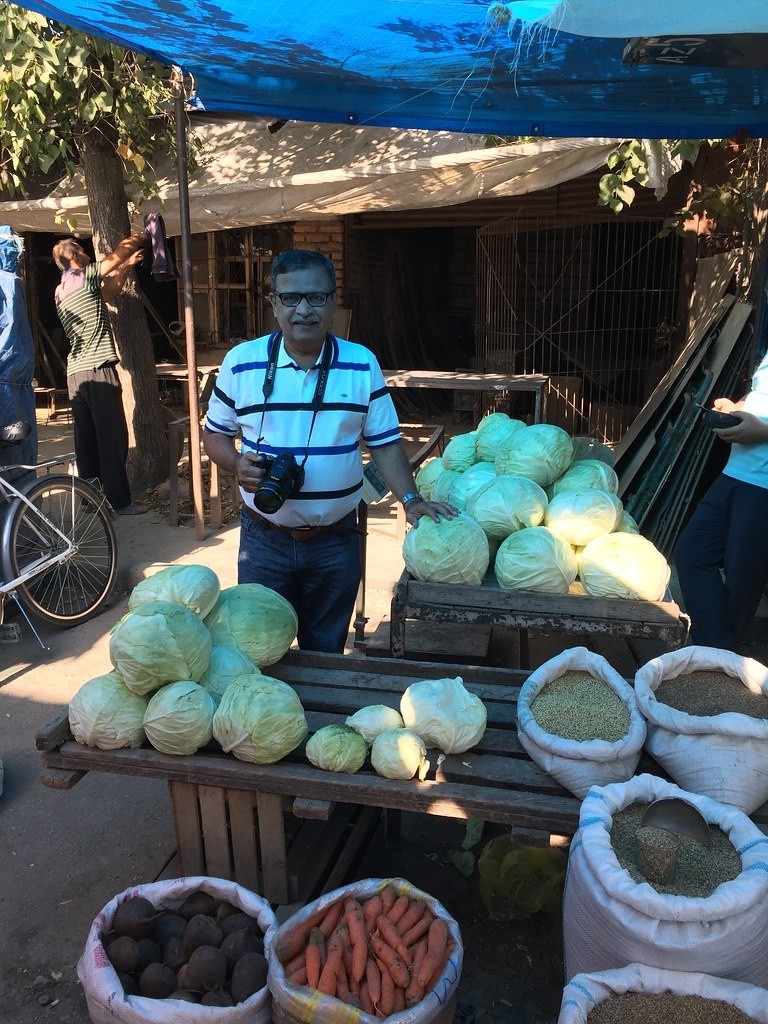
[401,492,426,505]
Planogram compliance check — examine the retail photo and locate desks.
[388,562,686,659]
[34,651,768,907]
[34,388,56,415]
[156,362,551,425]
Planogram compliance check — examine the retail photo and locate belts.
[244,504,357,542]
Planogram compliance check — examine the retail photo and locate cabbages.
[402,412,672,603]
[66,564,487,782]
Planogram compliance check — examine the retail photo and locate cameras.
[252,451,305,514]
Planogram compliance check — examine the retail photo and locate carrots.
[276,887,452,1018]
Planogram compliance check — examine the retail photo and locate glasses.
[274,288,337,307]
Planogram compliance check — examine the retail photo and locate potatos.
[104,891,269,1007]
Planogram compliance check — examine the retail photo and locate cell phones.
[695,403,709,412]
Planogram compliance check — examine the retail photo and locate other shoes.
[117,502,148,515]
[79,505,96,513]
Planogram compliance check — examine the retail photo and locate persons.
[201,249,461,655]
[672,351,767,652]
[51,232,148,516]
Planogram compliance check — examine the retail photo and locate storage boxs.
[547,375,582,434]
[591,401,631,440]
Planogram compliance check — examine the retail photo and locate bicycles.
[0,421,117,654]
